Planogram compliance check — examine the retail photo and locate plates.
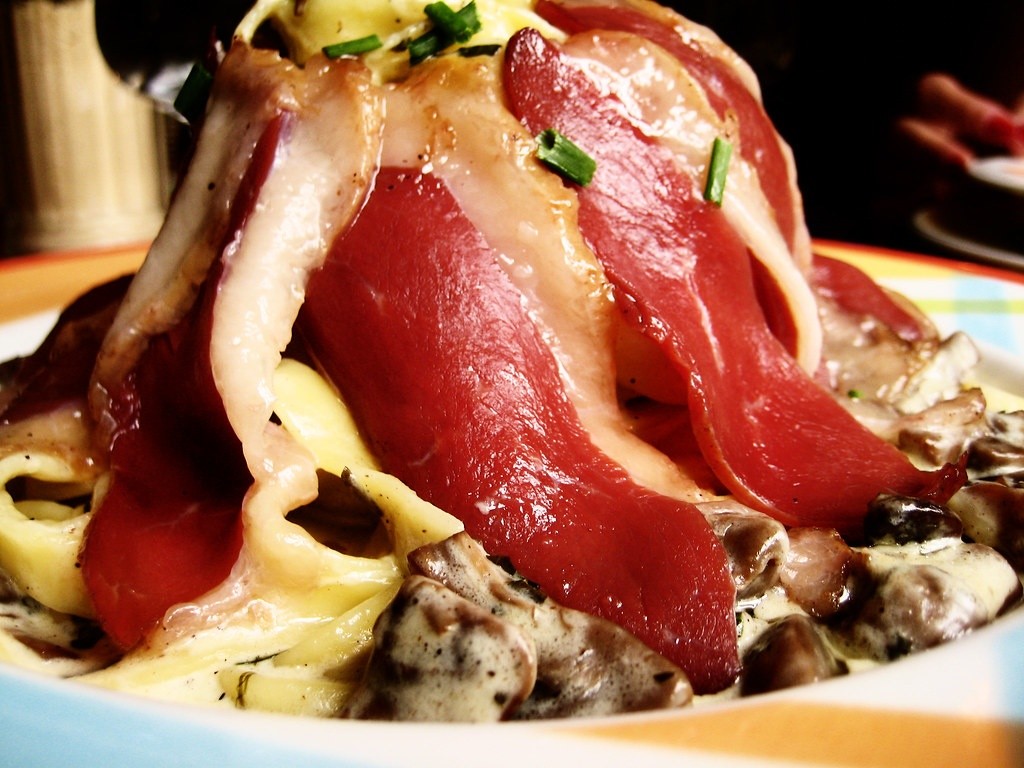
[914,204,1024,265]
[0,240,1024,767]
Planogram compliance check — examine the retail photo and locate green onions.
[317,0,731,207]
